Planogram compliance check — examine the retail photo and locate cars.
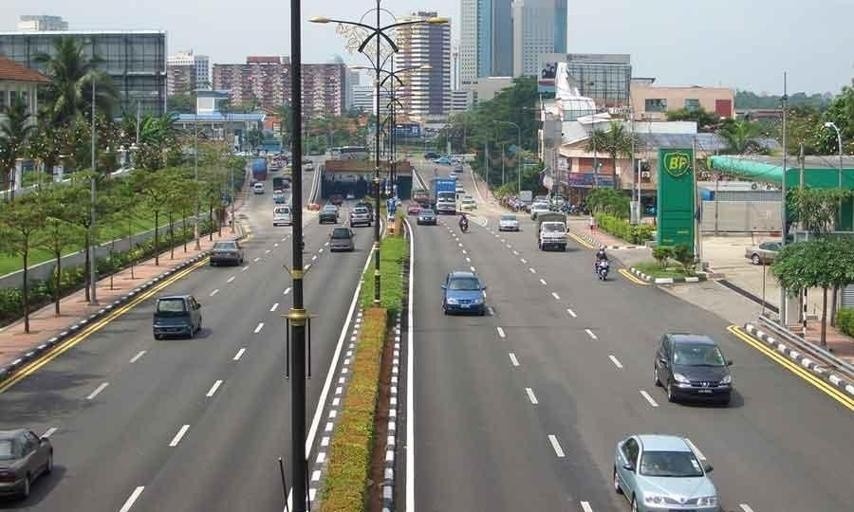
[505,191,581,220]
[745,241,782,267]
[319,189,374,227]
[0,428,52,501]
[612,435,720,512]
[498,213,520,232]
[236,149,313,226]
[409,152,476,225]
[440,271,486,317]
[653,331,733,406]
[209,241,245,265]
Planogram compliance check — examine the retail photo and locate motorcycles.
[594,257,612,280]
[460,220,468,232]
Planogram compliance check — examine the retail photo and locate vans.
[329,228,356,250]
[153,295,203,340]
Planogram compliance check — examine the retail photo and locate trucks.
[535,212,568,251]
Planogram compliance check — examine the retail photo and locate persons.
[595,247,609,264]
[589,214,596,236]
[459,214,468,225]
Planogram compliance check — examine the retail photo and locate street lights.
[492,120,522,191]
[308,2,450,307]
[819,122,843,231]
[347,65,433,237]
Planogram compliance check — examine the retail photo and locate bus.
[340,146,369,159]
